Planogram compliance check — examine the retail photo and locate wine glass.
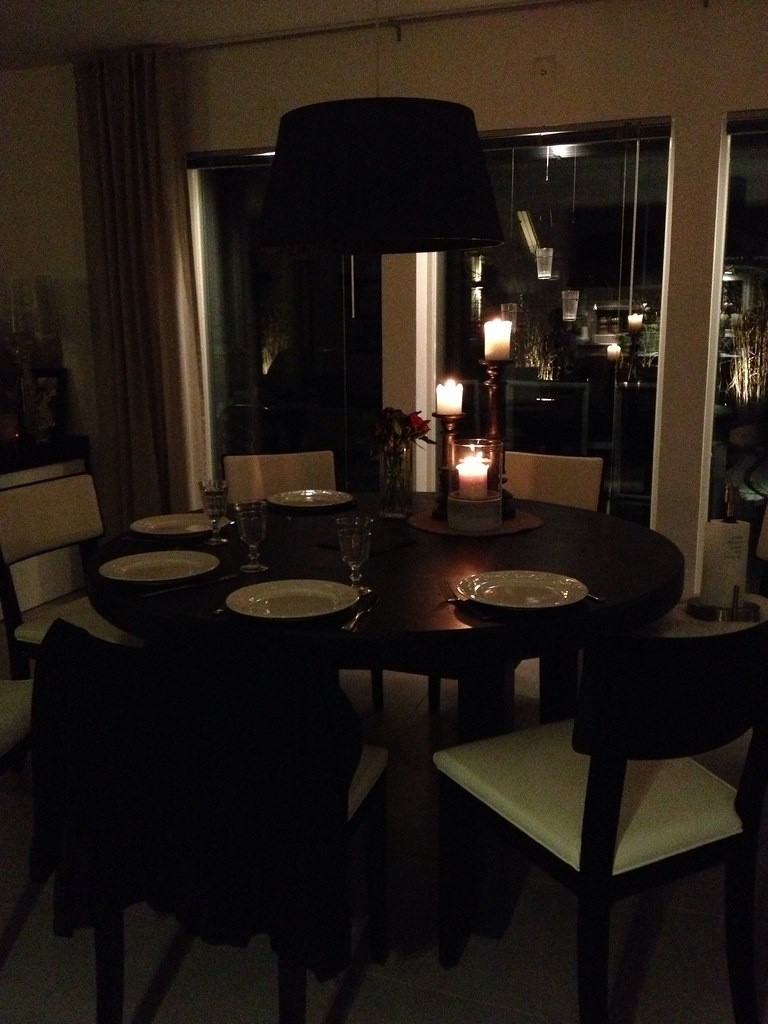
[336,515,375,596]
[234,499,268,575]
[199,480,228,545]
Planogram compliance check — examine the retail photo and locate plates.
[99,550,221,585]
[457,570,588,610]
[130,513,229,538]
[268,490,356,510]
[225,579,359,621]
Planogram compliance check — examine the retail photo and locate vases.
[377,441,416,518]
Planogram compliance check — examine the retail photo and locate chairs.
[504,378,590,457]
[428,449,610,726]
[431,616,768,1024]
[219,447,384,712]
[0,677,51,886]
[611,377,657,517]
[0,472,148,680]
[27,617,383,1024]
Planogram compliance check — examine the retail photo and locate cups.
[501,302,517,334]
[536,247,553,280]
[561,291,579,322]
[448,438,505,531]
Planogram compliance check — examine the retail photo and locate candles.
[628,313,643,334]
[605,344,621,360]
[435,378,463,415]
[483,319,512,360]
[456,456,488,500]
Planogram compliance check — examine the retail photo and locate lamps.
[560,146,580,322]
[500,149,517,334]
[537,146,553,279]
[255,0,506,256]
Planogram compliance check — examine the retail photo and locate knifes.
[139,574,240,598]
[340,594,381,631]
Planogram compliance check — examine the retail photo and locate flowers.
[369,406,438,513]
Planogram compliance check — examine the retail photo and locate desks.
[101,490,684,971]
